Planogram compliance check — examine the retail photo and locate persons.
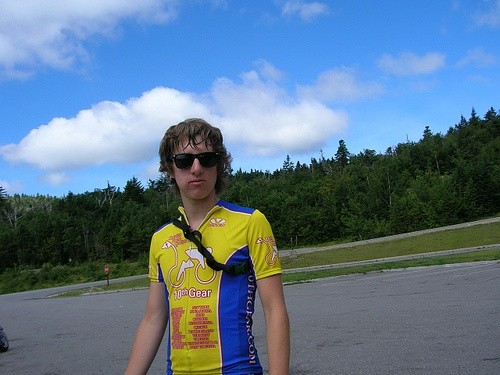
[123,118,291,375]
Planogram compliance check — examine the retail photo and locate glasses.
[170,152,220,169]
